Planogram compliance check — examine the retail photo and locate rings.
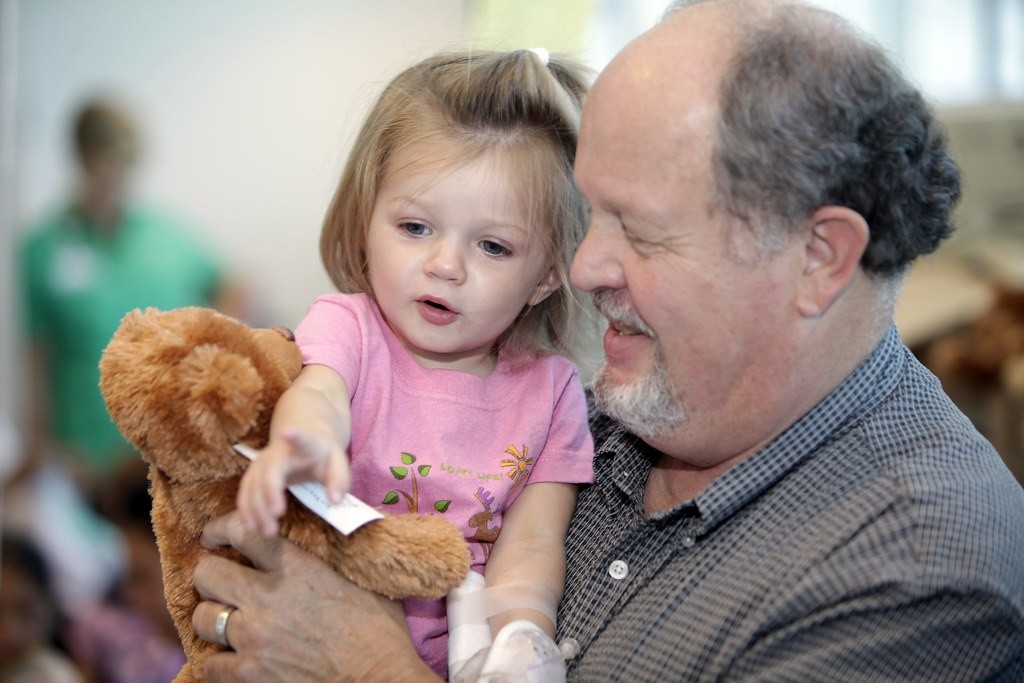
[214,605,237,646]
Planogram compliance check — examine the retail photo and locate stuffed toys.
[97,305,474,683]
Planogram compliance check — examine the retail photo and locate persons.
[12,99,248,492]
[192,0,1024,683]
[0,535,85,683]
